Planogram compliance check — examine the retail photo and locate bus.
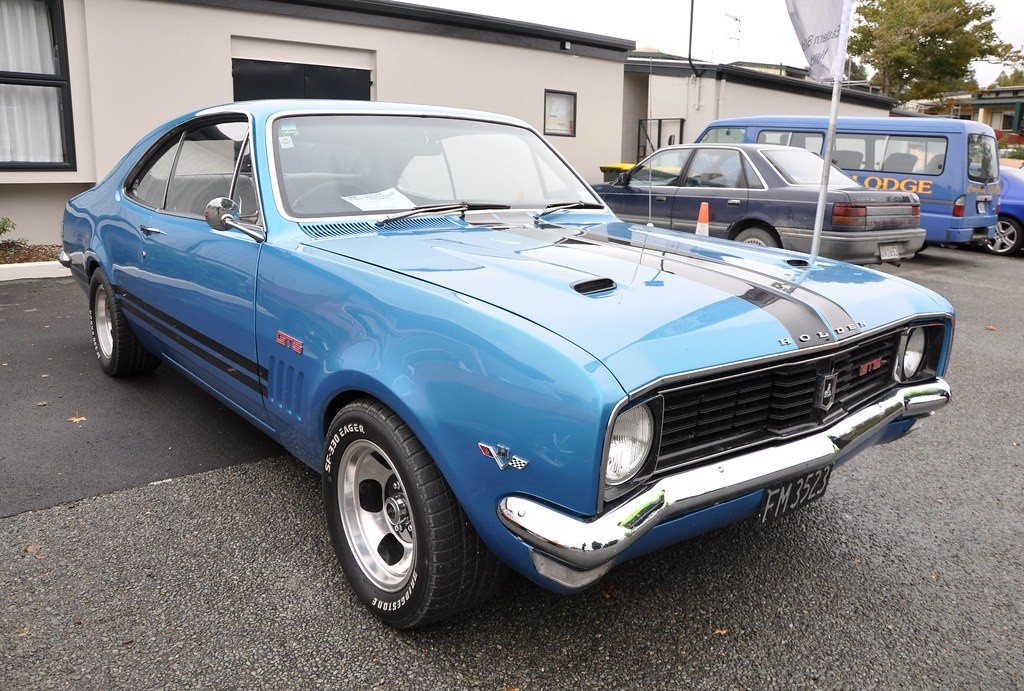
[691,115,1001,254]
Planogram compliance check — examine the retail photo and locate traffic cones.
[694,201,710,236]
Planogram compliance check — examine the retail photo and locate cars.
[548,141,928,268]
[59,96,959,630]
[915,163,1024,256]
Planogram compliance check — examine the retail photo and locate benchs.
[924,155,973,174]
[831,150,863,169]
[757,133,804,148]
[138,174,389,212]
[883,153,917,172]
